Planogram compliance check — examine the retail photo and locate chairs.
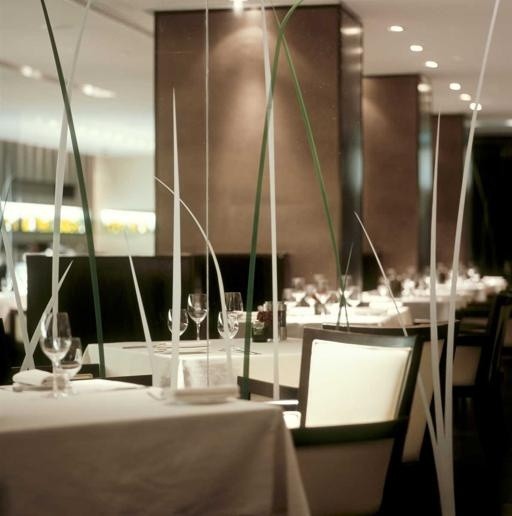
[82,336,302,400]
[235,325,425,516]
[9,364,101,383]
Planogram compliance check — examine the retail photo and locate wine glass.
[39,311,83,404]
[284,271,360,320]
[165,292,243,352]
